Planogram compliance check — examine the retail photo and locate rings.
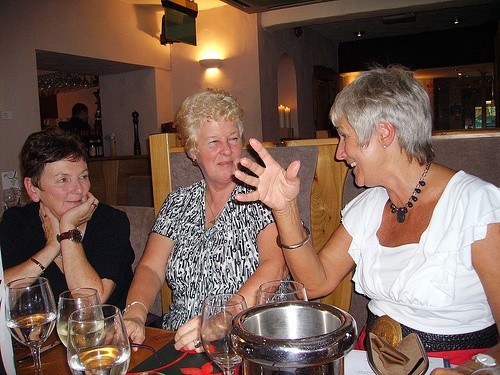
[44,215,47,218]
[193,340,202,348]
[92,203,96,210]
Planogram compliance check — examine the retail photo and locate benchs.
[149,129,500,347]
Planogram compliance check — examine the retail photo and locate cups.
[56,288,104,347]
[4,188,17,208]
[257,280,308,305]
[67,305,131,375]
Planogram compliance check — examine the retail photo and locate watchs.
[472,353,496,366]
[57,229,82,243]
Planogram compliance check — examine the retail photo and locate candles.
[285,107,291,128]
[278,104,285,128]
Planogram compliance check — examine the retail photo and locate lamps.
[199,59,221,68]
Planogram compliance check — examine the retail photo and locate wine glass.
[5,277,57,375]
[201,293,247,375]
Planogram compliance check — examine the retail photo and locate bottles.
[111,136,116,155]
[88,138,103,158]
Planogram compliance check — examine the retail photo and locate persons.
[100,88,300,359]
[67,103,91,143]
[235,67,500,375]
[0,126,135,321]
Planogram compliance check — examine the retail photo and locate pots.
[227,301,358,375]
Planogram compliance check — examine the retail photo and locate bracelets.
[123,301,148,315]
[30,257,45,272]
[276,219,310,248]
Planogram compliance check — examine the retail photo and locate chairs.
[109,204,163,318]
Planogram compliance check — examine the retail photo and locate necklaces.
[391,160,432,222]
[206,198,224,225]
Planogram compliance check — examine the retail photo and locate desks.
[0,318,349,375]
